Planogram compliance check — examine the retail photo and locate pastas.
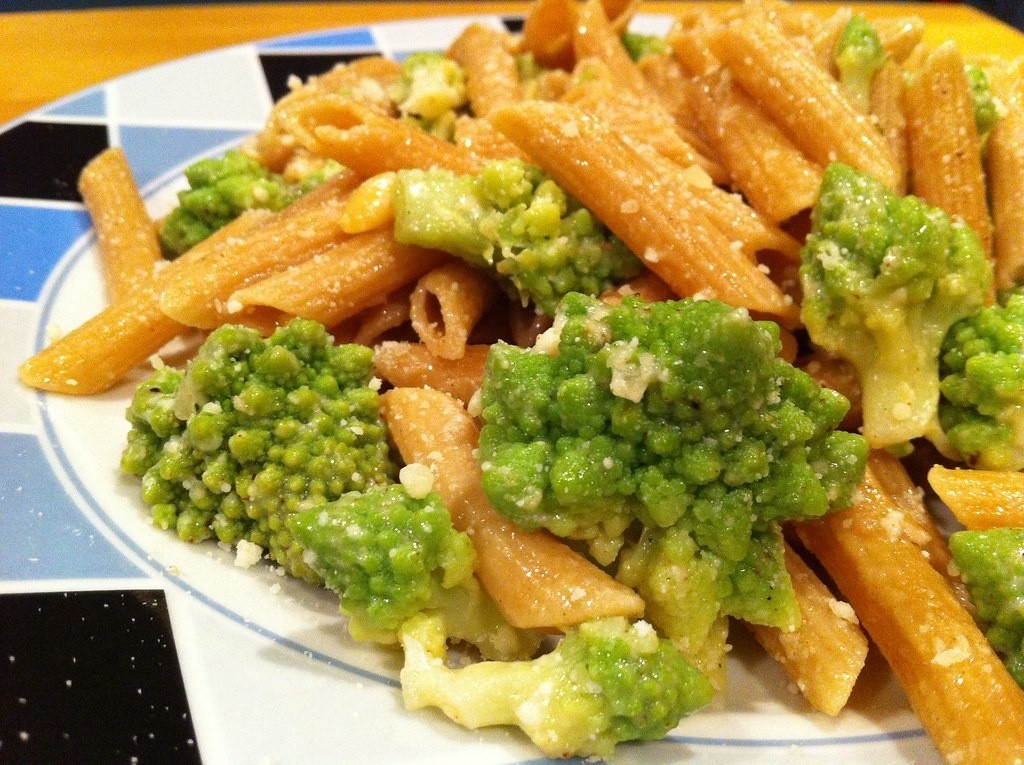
[21,0,1024,765]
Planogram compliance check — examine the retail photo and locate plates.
[0,15,943,765]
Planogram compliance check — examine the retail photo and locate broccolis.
[119,21,1024,765]
